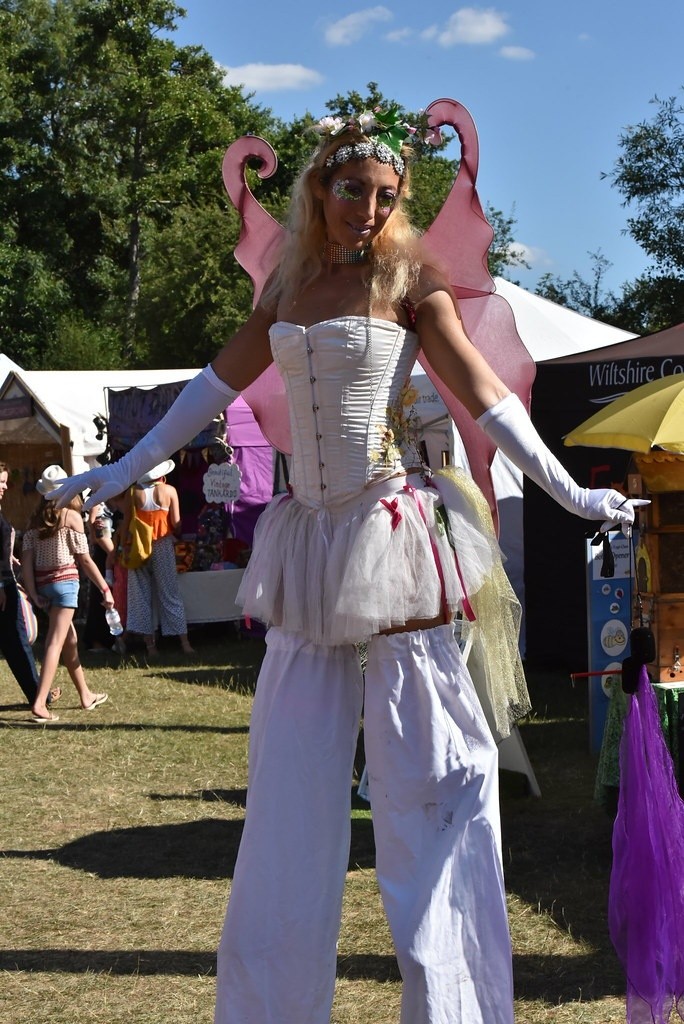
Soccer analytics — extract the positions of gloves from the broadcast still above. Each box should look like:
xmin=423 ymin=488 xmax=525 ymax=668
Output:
xmin=43 ymin=362 xmax=241 ymax=512
xmin=475 ymin=392 xmax=652 ymax=540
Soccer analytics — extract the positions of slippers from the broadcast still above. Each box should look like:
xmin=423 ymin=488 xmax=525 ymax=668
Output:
xmin=32 ymin=713 xmax=60 ymax=723
xmin=82 ymin=692 xmax=108 ymax=710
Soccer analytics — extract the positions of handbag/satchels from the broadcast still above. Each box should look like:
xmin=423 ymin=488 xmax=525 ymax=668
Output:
xmin=119 ymin=485 xmax=152 ymax=569
xmin=9 ymin=526 xmax=38 ymax=645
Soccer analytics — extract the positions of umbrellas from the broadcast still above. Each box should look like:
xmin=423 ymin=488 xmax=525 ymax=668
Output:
xmin=561 ymin=372 xmax=684 ymax=455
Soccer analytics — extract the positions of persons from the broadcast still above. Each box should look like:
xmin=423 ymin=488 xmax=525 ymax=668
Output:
xmin=88 ymin=459 xmax=197 ymax=656
xmin=44 ymin=99 xmax=651 ymax=1023
xmin=22 ymin=465 xmax=114 ymax=723
xmin=0 ymin=462 xmax=63 ymax=708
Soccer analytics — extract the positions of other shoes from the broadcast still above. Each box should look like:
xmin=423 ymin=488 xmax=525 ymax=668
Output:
xmin=49 ymin=687 xmax=63 ymax=703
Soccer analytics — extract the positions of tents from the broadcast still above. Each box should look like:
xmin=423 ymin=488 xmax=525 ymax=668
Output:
xmin=1 ymin=276 xmax=642 ymax=623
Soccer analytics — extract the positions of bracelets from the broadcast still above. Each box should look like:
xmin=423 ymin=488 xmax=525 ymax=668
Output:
xmin=101 ymin=587 xmax=109 ymax=594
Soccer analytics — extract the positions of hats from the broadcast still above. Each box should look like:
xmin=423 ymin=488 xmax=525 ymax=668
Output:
xmin=137 ymin=459 xmax=177 ymax=483
xmin=35 ymin=465 xmax=68 ymax=497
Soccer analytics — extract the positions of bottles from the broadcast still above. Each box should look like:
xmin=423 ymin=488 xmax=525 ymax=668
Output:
xmin=105 ymin=607 xmax=123 ymax=635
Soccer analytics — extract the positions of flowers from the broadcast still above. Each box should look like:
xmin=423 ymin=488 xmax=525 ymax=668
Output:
xmin=316 ymin=82 xmax=444 ymax=155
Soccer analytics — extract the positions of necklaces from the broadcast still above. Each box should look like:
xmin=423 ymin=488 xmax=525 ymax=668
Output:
xmin=326 ymin=243 xmax=370 ymax=263
